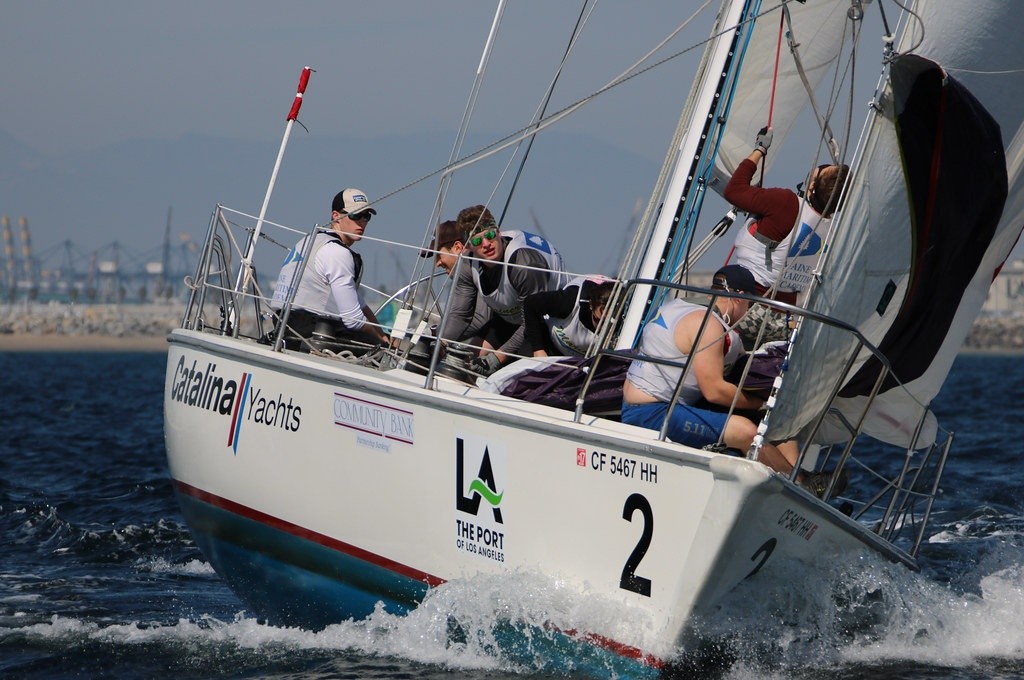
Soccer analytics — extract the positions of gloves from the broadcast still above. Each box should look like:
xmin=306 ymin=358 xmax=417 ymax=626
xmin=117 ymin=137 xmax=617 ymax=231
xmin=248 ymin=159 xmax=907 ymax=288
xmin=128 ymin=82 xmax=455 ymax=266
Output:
xmin=430 ymin=341 xmax=448 ymax=363
xmin=469 ymin=352 xmax=502 ymax=376
xmin=753 ymin=126 xmax=773 ymax=156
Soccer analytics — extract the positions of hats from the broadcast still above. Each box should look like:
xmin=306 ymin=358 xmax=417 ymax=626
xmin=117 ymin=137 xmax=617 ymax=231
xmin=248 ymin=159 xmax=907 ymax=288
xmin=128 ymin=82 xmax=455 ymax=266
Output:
xmin=418 ymin=220 xmax=463 ymax=258
xmin=332 ymin=188 xmax=377 ymax=216
xmin=713 ymin=265 xmax=756 ymax=295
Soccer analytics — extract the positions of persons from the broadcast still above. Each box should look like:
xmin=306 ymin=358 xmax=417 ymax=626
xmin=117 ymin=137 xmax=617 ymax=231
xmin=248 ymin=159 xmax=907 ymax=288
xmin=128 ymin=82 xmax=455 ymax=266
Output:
xmin=523 ymin=274 xmax=630 ymax=361
xmin=431 ymin=204 xmax=573 ymax=378
xmin=418 ymin=219 xmax=493 ymax=356
xmin=724 ymin=125 xmax=854 ymax=353
xmin=270 ymin=188 xmax=393 ymax=347
xmin=618 ymin=262 xmax=807 ymax=484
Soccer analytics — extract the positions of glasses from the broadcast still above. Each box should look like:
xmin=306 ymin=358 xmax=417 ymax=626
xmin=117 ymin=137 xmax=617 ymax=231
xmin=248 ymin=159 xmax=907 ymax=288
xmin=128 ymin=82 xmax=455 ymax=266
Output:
xmin=471 ymin=229 xmax=496 ymax=247
xmin=816 ymin=164 xmax=831 ymax=179
xmin=341 ymin=211 xmax=371 ymax=222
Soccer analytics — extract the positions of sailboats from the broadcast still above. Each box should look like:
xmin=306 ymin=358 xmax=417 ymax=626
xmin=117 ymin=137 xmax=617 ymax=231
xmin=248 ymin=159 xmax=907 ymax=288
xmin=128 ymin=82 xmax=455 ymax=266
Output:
xmin=164 ymin=0 xmax=1024 ymax=680
